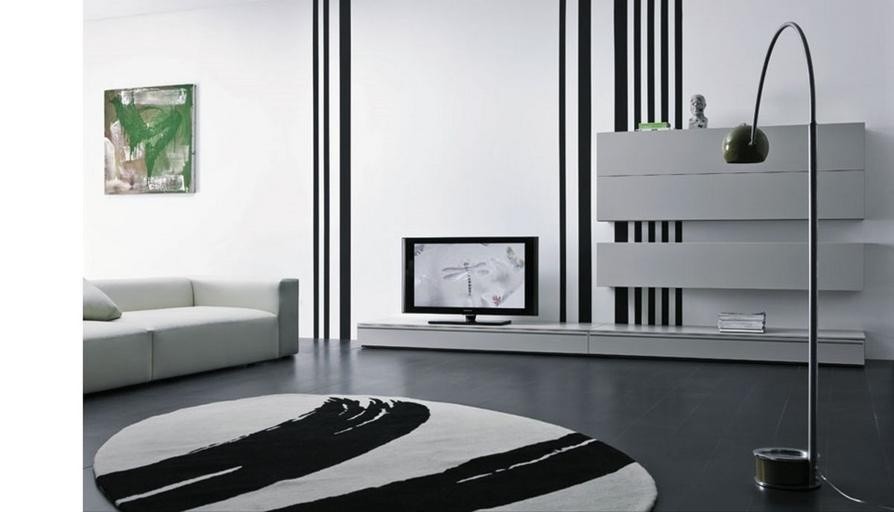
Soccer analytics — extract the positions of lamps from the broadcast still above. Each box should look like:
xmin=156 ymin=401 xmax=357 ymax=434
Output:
xmin=723 ymin=19 xmax=816 ymax=491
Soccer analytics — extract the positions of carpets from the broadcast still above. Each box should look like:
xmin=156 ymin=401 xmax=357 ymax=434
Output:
xmin=92 ymin=393 xmax=661 ymax=511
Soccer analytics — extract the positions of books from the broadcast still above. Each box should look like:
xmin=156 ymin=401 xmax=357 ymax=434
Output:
xmin=715 ymin=311 xmax=766 ymax=334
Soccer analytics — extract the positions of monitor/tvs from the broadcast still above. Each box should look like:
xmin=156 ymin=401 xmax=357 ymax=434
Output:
xmin=400 ymin=235 xmax=540 ymax=325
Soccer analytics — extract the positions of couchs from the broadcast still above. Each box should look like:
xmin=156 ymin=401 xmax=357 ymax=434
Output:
xmin=84 ymin=273 xmax=298 ymax=394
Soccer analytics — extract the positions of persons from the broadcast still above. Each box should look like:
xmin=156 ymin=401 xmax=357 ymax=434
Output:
xmin=687 ymin=95 xmax=708 ymax=128
